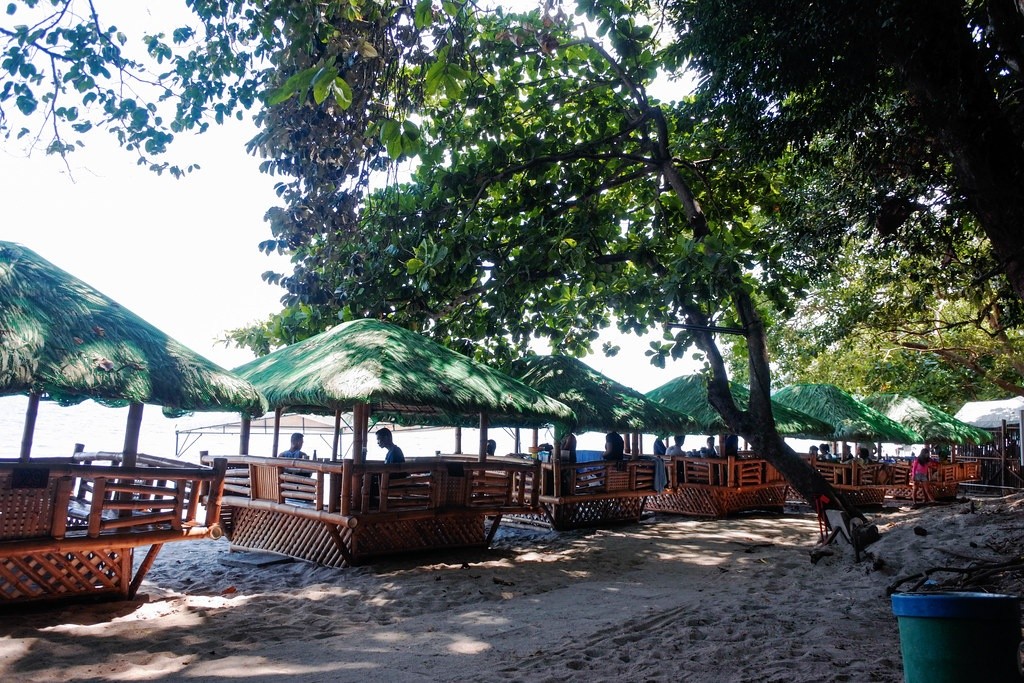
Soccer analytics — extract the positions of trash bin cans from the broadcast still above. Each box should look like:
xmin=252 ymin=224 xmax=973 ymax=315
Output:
xmin=890 ymin=591 xmax=1024 ymax=683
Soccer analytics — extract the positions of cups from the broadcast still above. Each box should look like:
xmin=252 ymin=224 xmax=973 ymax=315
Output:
xmin=536 ymin=451 xmax=544 ymax=462
xmin=692 ymin=449 xmax=696 ymax=455
xmin=317 ymin=458 xmax=323 ymax=463
xmin=323 ymin=458 xmax=330 ymax=464
xmin=532 ymin=452 xmax=537 ymax=459
xmin=542 ymin=451 xmax=550 ymax=463
xmin=302 ymin=455 xmax=309 ymax=460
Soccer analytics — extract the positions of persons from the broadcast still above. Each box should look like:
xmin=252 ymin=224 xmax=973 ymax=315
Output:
xmin=368 ymin=427 xmax=408 ymax=510
xmin=910 ymin=447 xmax=938 ymax=506
xmin=485 ymin=439 xmax=497 ymax=456
xmin=724 ymin=434 xmax=739 ymax=459
xmin=919 ymin=448 xmax=938 ymax=505
xmin=601 ymin=429 xmax=624 ymax=460
xmin=559 ymin=432 xmax=577 ymax=464
xmin=705 ymin=436 xmax=720 ymax=459
xmin=935 ymin=445 xmax=952 ymax=464
xmin=665 ymin=435 xmax=686 ymax=456
xmin=806 ymin=437 xmax=879 ymax=465
xmin=653 ymin=436 xmax=667 ymax=456
xmin=932 ymin=451 xmax=949 ymax=482
xmin=277 ymin=432 xmax=310 ymax=475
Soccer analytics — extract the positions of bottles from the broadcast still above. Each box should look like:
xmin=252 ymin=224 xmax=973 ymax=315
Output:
xmin=312 ymin=450 xmax=317 ymax=461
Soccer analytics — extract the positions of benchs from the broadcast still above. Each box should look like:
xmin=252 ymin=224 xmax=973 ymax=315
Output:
xmin=67 ymin=506 xmax=108 ymax=528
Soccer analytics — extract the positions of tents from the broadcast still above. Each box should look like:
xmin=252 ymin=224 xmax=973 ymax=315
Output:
xmin=339 ymin=410 xmax=522 ymax=461
xmin=173 ymin=410 xmax=342 ymax=461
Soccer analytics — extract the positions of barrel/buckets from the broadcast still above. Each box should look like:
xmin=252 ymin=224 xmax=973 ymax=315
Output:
xmin=891 ymin=591 xmax=1022 ymax=683
